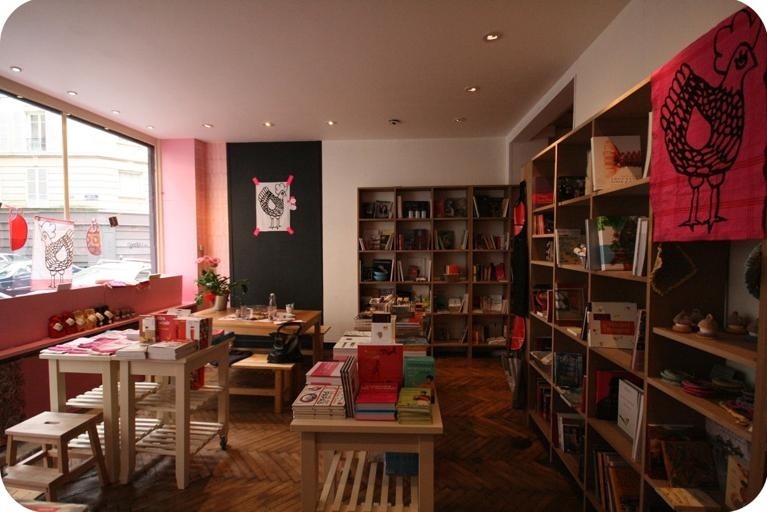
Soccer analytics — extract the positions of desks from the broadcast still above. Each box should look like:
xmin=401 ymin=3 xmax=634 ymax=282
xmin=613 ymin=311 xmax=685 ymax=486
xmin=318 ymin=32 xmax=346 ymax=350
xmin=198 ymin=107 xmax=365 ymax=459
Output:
xmin=39 ymin=331 xmax=120 ymax=483
xmin=190 ymin=304 xmax=323 ymax=386
xmin=292 ymin=325 xmax=441 ymax=511
xmin=118 ymin=331 xmax=234 ymax=490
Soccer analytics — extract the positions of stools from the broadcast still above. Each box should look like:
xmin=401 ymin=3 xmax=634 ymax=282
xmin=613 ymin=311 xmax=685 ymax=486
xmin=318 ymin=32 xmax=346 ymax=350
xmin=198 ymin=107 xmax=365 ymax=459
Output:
xmin=227 ymin=352 xmax=296 ymax=414
xmin=5 ymin=411 xmax=107 ymax=511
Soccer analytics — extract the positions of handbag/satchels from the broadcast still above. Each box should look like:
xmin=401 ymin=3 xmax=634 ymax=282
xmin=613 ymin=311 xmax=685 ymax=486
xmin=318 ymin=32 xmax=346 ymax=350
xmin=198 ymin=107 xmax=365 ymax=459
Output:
xmin=267 ymin=332 xmax=304 ymax=364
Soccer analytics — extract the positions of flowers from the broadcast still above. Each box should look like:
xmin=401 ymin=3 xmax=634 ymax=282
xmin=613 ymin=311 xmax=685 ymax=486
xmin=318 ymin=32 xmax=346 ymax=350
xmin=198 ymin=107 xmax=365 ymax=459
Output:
xmin=192 ymin=255 xmax=250 ymax=309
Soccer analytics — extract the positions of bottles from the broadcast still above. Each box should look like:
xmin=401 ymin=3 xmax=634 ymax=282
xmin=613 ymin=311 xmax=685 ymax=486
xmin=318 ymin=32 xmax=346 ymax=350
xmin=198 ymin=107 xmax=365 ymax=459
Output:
xmin=399 ymin=230 xmax=426 ymax=250
xmin=234 ymin=292 xmax=296 ymax=325
xmin=47 ymin=304 xmax=135 ymax=336
xmin=409 ymin=207 xmax=427 ymax=218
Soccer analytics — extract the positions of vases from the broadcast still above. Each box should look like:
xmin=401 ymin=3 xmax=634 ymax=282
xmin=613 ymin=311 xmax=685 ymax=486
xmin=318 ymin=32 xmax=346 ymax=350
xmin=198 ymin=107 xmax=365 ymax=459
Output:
xmin=213 ymin=293 xmax=229 ymax=310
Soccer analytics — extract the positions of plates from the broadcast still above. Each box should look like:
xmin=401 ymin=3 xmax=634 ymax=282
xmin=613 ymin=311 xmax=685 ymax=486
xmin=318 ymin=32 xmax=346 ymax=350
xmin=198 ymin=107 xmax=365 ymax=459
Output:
xmin=658 ymin=364 xmax=755 ymax=406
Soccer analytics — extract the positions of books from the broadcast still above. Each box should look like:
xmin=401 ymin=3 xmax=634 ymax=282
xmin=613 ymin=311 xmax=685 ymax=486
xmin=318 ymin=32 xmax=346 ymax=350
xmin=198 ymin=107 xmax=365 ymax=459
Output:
xmin=586 ymin=302 xmax=636 ymax=348
xmin=585 ymin=219 xmax=601 ymax=271
xmin=706 ymin=421 xmax=753 ymax=508
xmin=473 ymin=232 xmax=496 ymax=249
xmin=472 ymin=197 xmax=479 ymax=218
xmin=595 ymin=371 xmax=623 ymax=421
xmin=554 ymin=352 xmax=582 ymax=388
xmin=473 ymin=324 xmax=490 ymax=344
xmin=547 ymin=290 xmax=553 ymax=323
xmin=473 ymin=264 xmax=494 ymax=282
xmin=533 ymin=213 xmax=553 ymax=234
xmin=648 ymin=424 xmax=713 ymax=488
xmin=643 ymin=113 xmax=653 ymax=179
xmin=287 ymin=327 xmax=438 ymax=427
xmin=633 ymin=218 xmax=649 ymax=276
xmin=389 ymin=258 xmax=404 ymax=283
xmin=617 ymin=379 xmax=644 ymax=462
xmin=597 ymin=215 xmax=640 ymax=271
xmin=591 ymin=136 xmax=642 ymax=191
xmin=456 ymin=320 xmax=469 ymax=344
xmin=434 ymin=229 xmax=454 ymax=250
xmin=353 ymin=195 xmax=513 ymax=344
xmin=554 ymin=288 xmax=585 ymax=327
xmin=591 ymin=452 xmax=640 ymax=510
xmin=39 ymin=305 xmax=235 ymax=362
xmin=458 ymin=293 xmax=469 ymax=313
xmin=397 ymin=197 xmax=402 ymax=218
xmin=557 ymin=414 xmax=586 ymax=454
xmin=359 ymin=228 xmax=395 ymax=250
xmin=397 ymin=228 xmax=431 ymax=249
xmin=460 ymin=230 xmax=468 ymax=250
xmin=631 ymin=309 xmax=645 ymax=371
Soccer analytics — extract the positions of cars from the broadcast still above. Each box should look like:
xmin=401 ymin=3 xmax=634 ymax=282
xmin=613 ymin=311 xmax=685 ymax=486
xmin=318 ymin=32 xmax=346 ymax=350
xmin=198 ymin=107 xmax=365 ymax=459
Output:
xmin=0 ymin=252 xmax=153 ymax=297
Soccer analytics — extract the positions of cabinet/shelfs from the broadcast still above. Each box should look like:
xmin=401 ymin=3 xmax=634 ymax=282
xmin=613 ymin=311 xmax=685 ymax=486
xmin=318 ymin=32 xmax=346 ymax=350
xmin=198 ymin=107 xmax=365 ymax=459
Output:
xmin=356 ymin=185 xmax=522 ymax=357
xmin=524 ymin=5 xmax=767 ymax=511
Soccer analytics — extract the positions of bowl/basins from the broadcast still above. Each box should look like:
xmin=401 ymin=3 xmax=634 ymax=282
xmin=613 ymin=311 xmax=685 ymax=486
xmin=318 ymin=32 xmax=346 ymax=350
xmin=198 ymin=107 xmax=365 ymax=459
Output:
xmin=374 ymin=272 xmax=386 ymax=282
